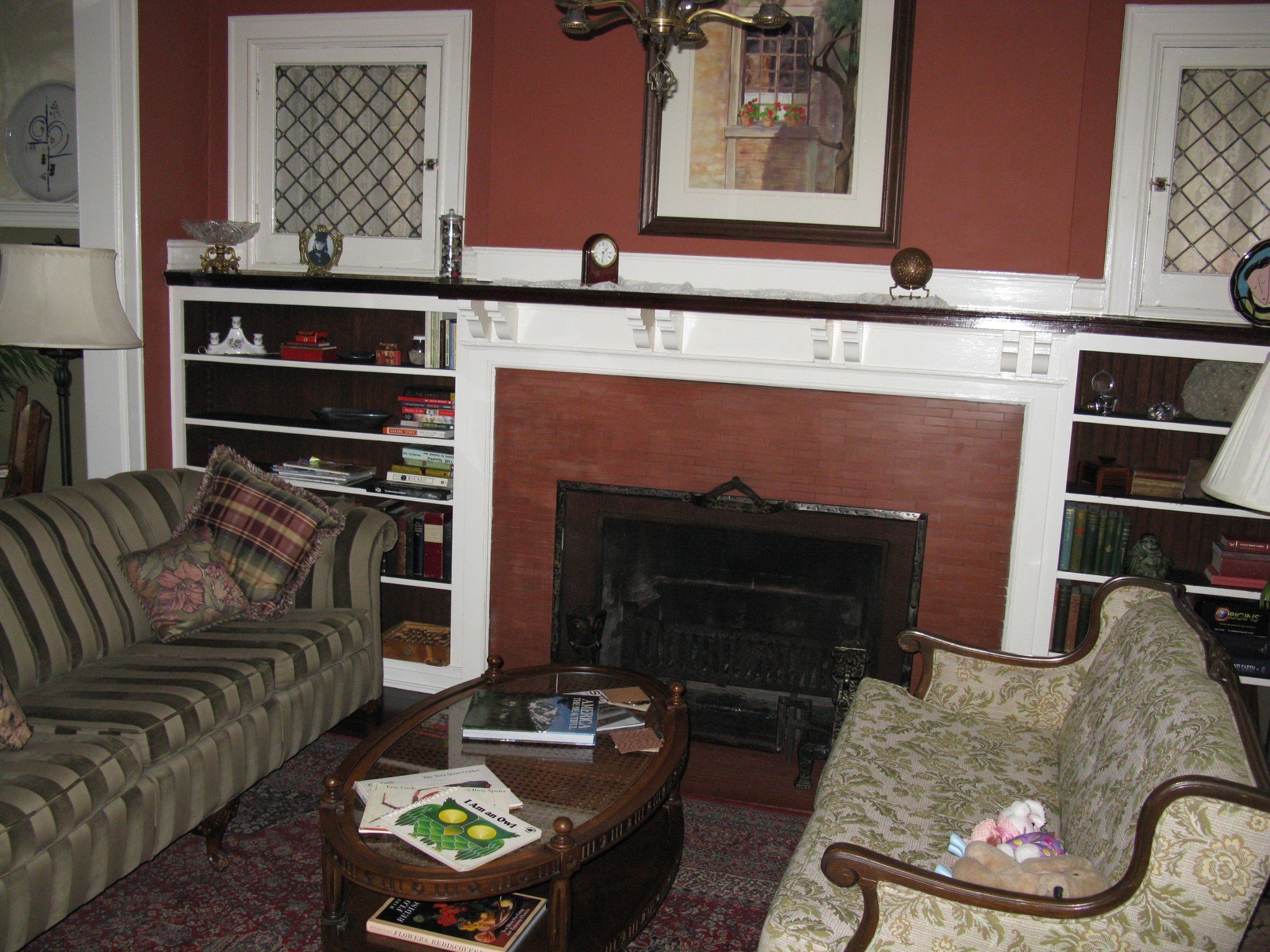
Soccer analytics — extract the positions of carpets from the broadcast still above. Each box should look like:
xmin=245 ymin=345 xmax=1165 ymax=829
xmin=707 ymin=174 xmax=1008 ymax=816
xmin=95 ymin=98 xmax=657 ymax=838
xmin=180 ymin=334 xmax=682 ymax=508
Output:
xmin=27 ymin=729 xmax=824 ymax=951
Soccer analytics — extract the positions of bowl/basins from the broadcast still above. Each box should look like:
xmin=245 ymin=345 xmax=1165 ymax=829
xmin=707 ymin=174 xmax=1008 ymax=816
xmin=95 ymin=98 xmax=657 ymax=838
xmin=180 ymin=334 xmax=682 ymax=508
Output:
xmin=310 ymin=405 xmax=389 ymax=429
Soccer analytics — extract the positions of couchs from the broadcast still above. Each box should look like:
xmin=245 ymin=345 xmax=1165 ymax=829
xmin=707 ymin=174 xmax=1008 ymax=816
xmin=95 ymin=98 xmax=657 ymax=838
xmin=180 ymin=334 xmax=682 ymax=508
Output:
xmin=747 ymin=579 xmax=1269 ymax=950
xmin=0 ymin=470 xmax=402 ymax=952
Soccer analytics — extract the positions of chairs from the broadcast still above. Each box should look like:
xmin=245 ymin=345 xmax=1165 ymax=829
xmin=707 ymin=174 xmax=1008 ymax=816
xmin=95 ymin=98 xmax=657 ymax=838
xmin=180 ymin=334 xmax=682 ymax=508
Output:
xmin=0 ymin=385 xmax=52 ymax=501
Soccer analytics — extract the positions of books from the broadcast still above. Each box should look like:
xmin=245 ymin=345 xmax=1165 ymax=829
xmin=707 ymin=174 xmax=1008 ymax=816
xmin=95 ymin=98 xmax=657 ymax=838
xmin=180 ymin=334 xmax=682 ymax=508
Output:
xmin=1047 ymin=444 xmax=1270 ymax=680
xmin=331 ymin=683 xmax=672 ymax=952
xmin=248 ymin=312 xmax=457 ymax=586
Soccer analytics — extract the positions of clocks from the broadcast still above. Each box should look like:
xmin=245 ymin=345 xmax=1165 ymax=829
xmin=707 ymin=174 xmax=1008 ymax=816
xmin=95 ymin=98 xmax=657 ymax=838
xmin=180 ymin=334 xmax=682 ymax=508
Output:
xmin=580 ymin=232 xmax=618 ymax=289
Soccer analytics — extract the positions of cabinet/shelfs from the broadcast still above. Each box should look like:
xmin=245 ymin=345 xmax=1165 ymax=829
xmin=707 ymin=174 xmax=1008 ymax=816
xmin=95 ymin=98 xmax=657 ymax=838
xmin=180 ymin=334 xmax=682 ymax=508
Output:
xmin=1038 ymin=346 xmax=1269 ymax=686
xmin=168 ymin=285 xmax=494 ymax=694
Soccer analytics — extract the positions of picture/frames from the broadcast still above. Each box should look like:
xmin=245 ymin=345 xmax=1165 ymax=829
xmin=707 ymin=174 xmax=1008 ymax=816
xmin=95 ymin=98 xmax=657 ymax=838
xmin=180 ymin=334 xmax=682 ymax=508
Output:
xmin=297 ymin=225 xmax=344 ymax=276
xmin=639 ymin=0 xmax=914 ymax=247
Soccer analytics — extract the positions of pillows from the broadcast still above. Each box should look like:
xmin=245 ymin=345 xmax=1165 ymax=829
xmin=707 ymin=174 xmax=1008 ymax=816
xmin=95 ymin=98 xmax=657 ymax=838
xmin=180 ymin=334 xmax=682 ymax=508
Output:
xmin=115 ymin=522 xmax=257 ymax=641
xmin=173 ymin=443 xmax=346 ymax=623
xmin=1 ymin=667 xmax=39 ymax=751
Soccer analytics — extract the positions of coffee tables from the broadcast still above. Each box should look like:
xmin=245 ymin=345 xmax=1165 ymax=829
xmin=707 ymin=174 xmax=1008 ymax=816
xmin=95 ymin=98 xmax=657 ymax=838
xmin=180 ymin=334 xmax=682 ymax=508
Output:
xmin=319 ymin=655 xmax=690 ymax=952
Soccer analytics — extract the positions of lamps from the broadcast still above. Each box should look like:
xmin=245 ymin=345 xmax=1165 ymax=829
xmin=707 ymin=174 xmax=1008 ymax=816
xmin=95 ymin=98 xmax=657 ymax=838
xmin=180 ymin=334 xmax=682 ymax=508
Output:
xmin=555 ymin=0 xmax=791 ymax=110
xmin=0 ymin=242 xmax=147 ymax=486
xmin=1201 ymin=358 xmax=1268 ymax=520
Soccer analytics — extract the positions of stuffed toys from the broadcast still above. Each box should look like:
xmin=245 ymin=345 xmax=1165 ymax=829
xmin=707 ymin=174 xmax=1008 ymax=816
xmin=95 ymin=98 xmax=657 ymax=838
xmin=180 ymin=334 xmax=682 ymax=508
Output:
xmin=931 ymin=794 xmax=1116 ymax=898
